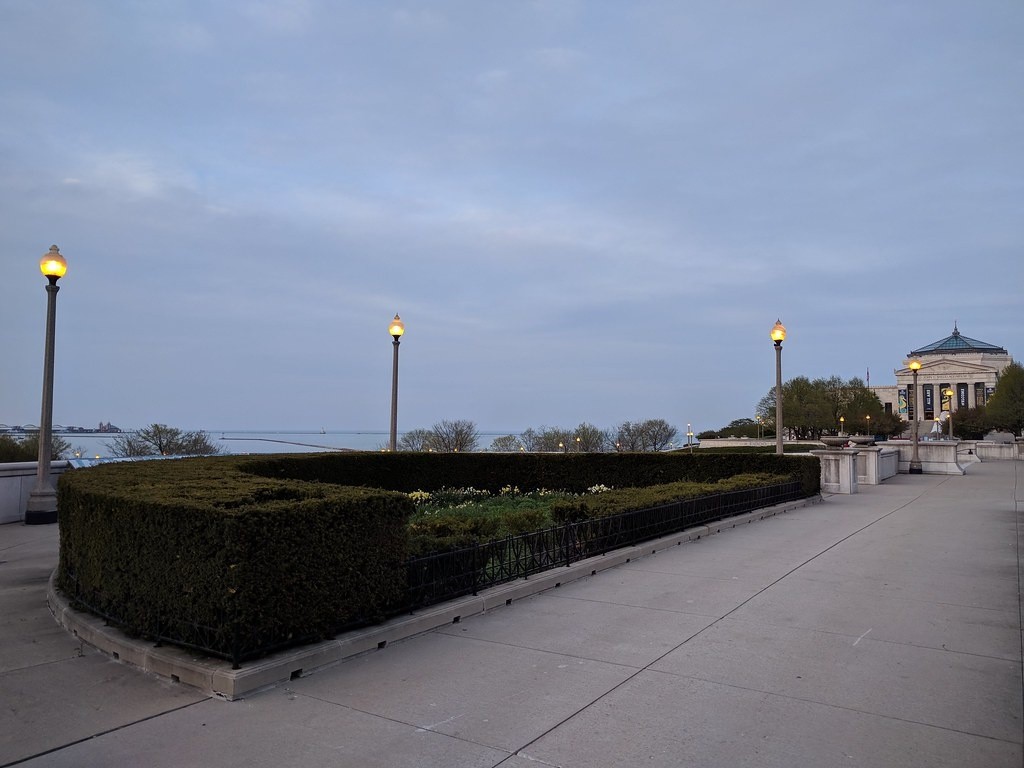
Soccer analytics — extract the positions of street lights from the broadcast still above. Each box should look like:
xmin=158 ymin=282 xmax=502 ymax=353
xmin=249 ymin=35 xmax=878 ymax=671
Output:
xmin=866 ymin=414 xmax=870 ymax=435
xmin=24 ymin=244 xmax=68 ymax=525
xmin=840 ymin=416 xmax=844 ymax=436
xmin=388 ymin=313 xmax=405 ymax=451
xmin=687 ymin=430 xmax=693 ymax=453
xmin=946 ymin=390 xmax=954 ymax=440
xmin=934 ymin=416 xmax=940 ymax=432
xmin=770 ymin=318 xmax=787 ymax=454
xmin=756 ymin=414 xmax=764 ymax=440
xmin=908 ymin=355 xmax=922 ymax=474
xmin=577 ymin=438 xmax=580 ymax=452
